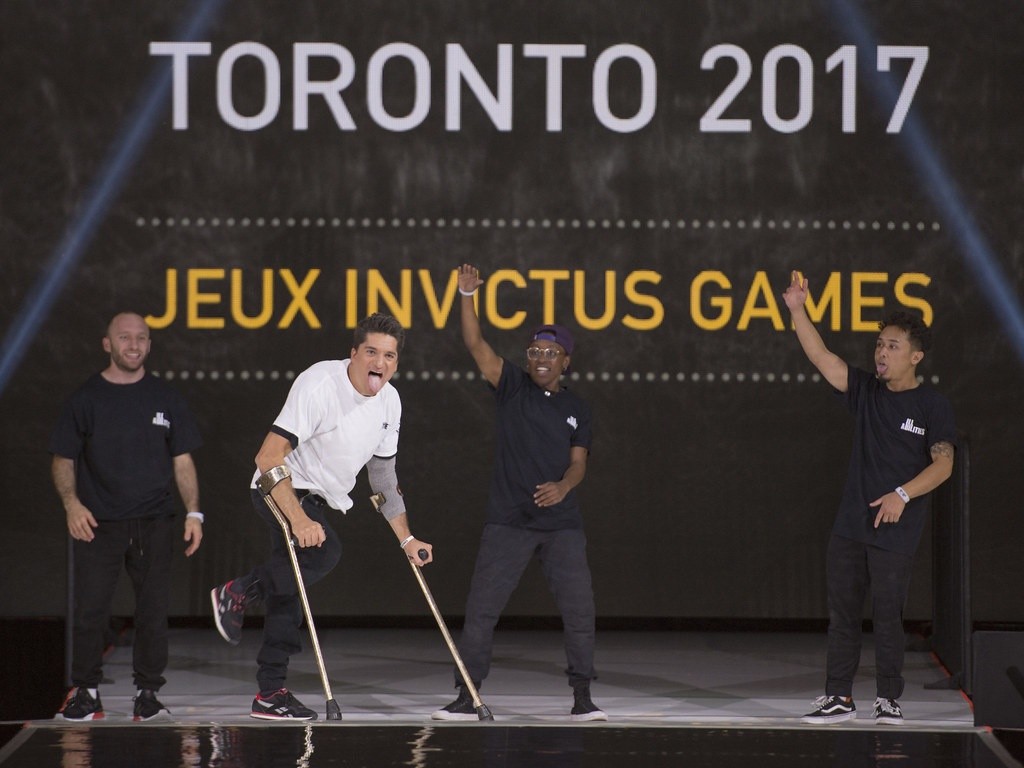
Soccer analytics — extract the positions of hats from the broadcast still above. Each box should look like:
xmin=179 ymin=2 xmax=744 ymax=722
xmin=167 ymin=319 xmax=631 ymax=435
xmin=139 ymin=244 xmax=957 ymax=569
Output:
xmin=528 ymin=325 xmax=575 ymax=376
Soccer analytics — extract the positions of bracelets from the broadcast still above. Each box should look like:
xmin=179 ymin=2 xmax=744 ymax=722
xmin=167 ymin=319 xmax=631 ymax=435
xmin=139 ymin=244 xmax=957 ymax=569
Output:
xmin=186 ymin=512 xmax=204 ymax=523
xmin=895 ymin=487 xmax=910 ymax=503
xmin=400 ymin=535 xmax=415 ymax=549
xmin=458 ymin=287 xmax=475 ymax=296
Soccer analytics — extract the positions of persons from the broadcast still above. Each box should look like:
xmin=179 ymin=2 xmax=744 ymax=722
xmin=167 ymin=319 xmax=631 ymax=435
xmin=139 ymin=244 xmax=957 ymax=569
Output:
xmin=211 ymin=313 xmax=433 ymax=721
xmin=784 ymin=271 xmax=955 ymax=725
xmin=48 ymin=311 xmax=204 ymax=723
xmin=431 ymin=263 xmax=609 ymax=721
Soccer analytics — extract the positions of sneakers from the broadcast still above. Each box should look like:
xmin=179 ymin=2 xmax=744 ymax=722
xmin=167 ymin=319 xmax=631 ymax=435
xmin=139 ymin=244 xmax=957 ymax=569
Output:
xmin=431 ymin=684 xmax=481 ymax=721
xmin=870 ymin=697 xmax=904 ymax=726
xmin=250 ymin=688 xmax=318 ymax=720
xmin=210 ymin=578 xmax=264 ymax=647
xmin=132 ymin=688 xmax=173 ymax=721
xmin=570 ymin=687 xmax=608 ymax=721
xmin=800 ymin=696 xmax=856 ymax=725
xmin=55 ymin=688 xmax=105 ymax=722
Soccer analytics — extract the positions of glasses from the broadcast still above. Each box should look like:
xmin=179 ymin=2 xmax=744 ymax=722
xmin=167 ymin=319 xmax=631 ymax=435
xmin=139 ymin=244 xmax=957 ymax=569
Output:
xmin=526 ymin=346 xmax=565 ymax=361
